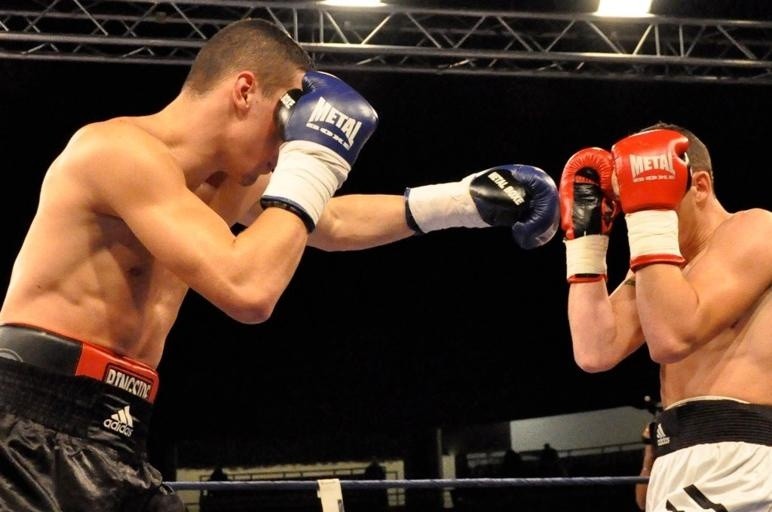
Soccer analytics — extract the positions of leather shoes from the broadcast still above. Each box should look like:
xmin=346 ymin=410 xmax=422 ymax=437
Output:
xmin=403 ymin=161 xmax=562 ymax=252
xmin=256 ymin=69 xmax=381 ymax=233
xmin=612 ymin=127 xmax=688 ymax=269
xmin=561 ymin=146 xmax=621 ymax=284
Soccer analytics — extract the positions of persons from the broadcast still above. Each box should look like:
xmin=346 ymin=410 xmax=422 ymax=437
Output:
xmin=499 ymin=448 xmax=521 ymax=468
xmin=560 ymin=122 xmax=772 ymax=512
xmin=364 ymin=456 xmax=388 ymax=479
xmin=0 ymin=17 xmax=562 ymax=510
xmin=209 ymin=463 xmax=227 ymax=481
